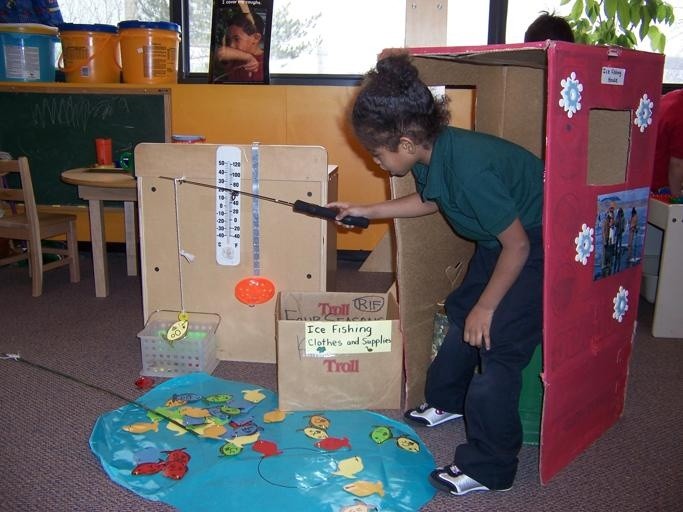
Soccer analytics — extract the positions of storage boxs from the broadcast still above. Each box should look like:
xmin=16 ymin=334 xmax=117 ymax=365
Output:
xmin=275 ymin=290 xmax=405 ymax=413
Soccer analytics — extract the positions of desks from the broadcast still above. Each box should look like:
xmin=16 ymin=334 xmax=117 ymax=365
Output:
xmin=646 ymin=196 xmax=681 ymax=340
xmin=61 ymin=166 xmax=143 ymax=298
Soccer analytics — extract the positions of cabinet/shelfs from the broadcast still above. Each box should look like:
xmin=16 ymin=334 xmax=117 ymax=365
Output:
xmin=168 ymin=83 xmax=476 ymax=262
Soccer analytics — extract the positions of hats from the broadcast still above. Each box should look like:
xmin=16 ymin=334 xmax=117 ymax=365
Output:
xmin=610 ymin=202 xmax=617 ymax=208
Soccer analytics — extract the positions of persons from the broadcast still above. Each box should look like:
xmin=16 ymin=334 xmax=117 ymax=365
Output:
xmin=217 ymin=13 xmax=264 ymax=82
xmin=651 ymin=90 xmax=682 ymax=201
xmin=525 ymin=10 xmax=574 ymax=45
xmin=325 ymin=53 xmax=544 ymax=497
xmin=600 ymin=202 xmax=637 ymax=248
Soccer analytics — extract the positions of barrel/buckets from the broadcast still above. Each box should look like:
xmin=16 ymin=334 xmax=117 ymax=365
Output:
xmin=0 ymin=23 xmax=57 ymax=83
xmin=59 ymin=22 xmax=119 ymax=84
xmin=118 ymin=20 xmax=181 ymax=84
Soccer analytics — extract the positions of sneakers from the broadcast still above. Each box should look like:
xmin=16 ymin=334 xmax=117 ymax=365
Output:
xmin=428 ymin=462 xmax=513 ymax=496
xmin=403 ymin=402 xmax=463 ymax=427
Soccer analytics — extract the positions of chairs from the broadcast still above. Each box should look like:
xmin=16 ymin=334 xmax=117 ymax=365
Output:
xmin=0 ymin=154 xmax=81 ymax=298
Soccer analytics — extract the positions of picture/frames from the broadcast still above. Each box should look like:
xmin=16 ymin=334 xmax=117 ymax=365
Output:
xmin=207 ymin=1 xmax=274 ymax=86
